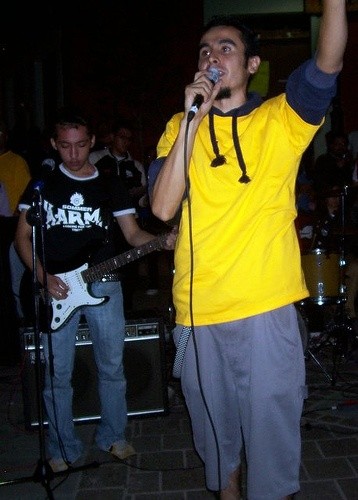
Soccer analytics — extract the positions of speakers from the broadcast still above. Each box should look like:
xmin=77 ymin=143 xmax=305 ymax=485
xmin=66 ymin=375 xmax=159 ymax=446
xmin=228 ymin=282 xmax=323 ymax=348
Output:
xmin=18 ymin=316 xmax=169 ymax=431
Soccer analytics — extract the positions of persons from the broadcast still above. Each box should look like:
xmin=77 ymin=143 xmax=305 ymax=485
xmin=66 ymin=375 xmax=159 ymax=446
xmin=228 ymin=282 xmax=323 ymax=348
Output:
xmin=14 ymin=112 xmax=180 ymax=477
xmin=295 ymin=127 xmax=358 ymax=319
xmin=0 ymin=123 xmax=174 ymax=367
xmin=147 ymin=0 xmax=347 ymax=500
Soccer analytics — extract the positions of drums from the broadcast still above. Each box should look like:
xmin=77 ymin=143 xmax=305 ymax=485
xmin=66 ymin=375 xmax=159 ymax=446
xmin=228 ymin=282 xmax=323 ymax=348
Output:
xmin=296 ymin=247 xmax=348 ymax=307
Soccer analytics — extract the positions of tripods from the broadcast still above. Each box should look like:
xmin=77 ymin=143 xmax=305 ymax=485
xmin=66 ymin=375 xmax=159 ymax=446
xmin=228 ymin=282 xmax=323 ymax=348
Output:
xmin=0 ymin=195 xmax=98 ymax=500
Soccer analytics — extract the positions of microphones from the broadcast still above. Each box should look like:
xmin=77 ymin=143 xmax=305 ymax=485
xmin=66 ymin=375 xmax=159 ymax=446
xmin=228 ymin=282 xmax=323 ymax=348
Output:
xmin=32 ymin=159 xmax=55 ymax=198
xmin=186 ymin=67 xmax=219 ymax=123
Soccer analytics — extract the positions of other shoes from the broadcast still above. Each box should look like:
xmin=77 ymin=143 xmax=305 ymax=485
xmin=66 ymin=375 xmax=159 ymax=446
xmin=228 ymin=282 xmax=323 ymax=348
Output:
xmin=111 ymin=440 xmax=136 ymax=460
xmin=43 ymin=457 xmax=73 ymax=471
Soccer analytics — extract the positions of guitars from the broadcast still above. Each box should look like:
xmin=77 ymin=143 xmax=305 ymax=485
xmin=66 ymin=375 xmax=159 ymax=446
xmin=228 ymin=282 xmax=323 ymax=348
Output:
xmin=19 ymin=229 xmax=180 ymax=334
